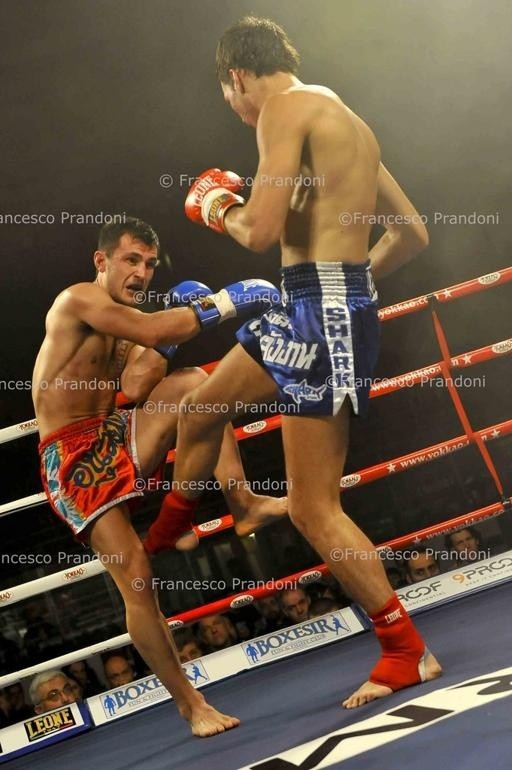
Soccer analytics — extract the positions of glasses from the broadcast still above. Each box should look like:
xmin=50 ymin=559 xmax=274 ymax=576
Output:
xmin=33 ymin=682 xmax=75 ymax=708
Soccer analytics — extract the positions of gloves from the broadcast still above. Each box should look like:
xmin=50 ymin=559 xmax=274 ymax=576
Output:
xmin=183 ymin=166 xmax=249 ymax=237
xmin=151 ymin=278 xmax=214 ymax=362
xmin=189 ymin=277 xmax=284 ymax=331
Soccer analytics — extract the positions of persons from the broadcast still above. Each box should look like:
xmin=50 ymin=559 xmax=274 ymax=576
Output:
xmin=0 ymin=527 xmax=489 ymax=727
xmin=35 ymin=213 xmax=289 ymax=738
xmin=139 ymin=14 xmax=442 ymax=710
